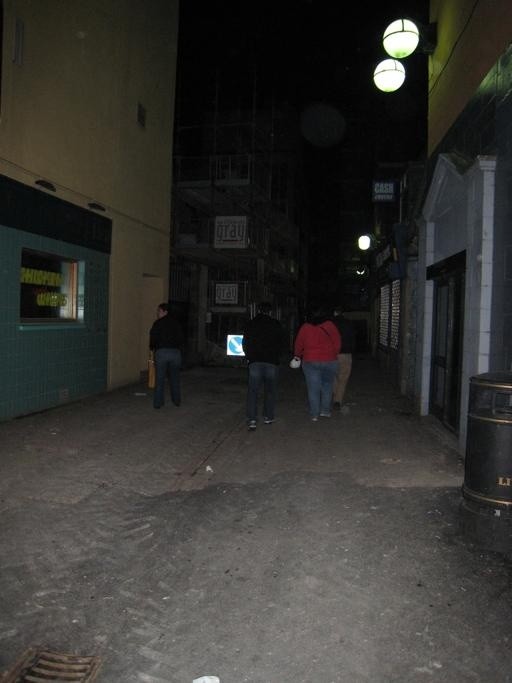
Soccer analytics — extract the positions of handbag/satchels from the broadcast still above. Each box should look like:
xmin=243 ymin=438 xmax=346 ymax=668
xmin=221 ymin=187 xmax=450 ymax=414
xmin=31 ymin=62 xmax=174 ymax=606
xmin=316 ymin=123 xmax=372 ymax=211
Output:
xmin=290 ymin=356 xmax=301 ymax=368
xmin=148 ymin=358 xmax=157 ymax=389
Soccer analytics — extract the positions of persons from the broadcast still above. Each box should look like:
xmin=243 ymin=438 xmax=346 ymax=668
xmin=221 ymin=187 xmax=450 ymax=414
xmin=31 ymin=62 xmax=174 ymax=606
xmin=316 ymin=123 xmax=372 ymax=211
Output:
xmin=333 ymin=314 xmax=355 ymax=410
xmin=148 ymin=302 xmax=184 ymax=409
xmin=241 ymin=300 xmax=285 ymax=430
xmin=292 ymin=305 xmax=343 ymax=422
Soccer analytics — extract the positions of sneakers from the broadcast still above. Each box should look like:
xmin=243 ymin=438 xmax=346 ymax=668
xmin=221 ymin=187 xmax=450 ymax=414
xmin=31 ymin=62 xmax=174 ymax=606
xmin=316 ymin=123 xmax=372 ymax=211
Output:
xmin=264 ymin=416 xmax=277 ymax=424
xmin=309 ymin=403 xmax=342 ymax=422
xmin=247 ymin=419 xmax=257 ymax=431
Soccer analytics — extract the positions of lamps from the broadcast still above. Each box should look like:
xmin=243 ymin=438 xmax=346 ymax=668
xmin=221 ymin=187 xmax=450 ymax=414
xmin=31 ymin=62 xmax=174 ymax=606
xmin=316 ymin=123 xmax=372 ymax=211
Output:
xmin=382 ymin=17 xmax=435 ymax=59
xmin=356 ymin=264 xmax=365 ymax=275
xmin=358 ymin=235 xmax=380 ymax=251
xmin=372 ymin=58 xmax=406 ymax=92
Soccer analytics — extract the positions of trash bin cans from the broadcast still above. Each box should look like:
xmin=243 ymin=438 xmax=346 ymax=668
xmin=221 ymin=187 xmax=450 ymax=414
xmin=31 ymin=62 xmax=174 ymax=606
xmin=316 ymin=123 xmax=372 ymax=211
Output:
xmin=460 ymin=371 xmax=512 ymax=554
